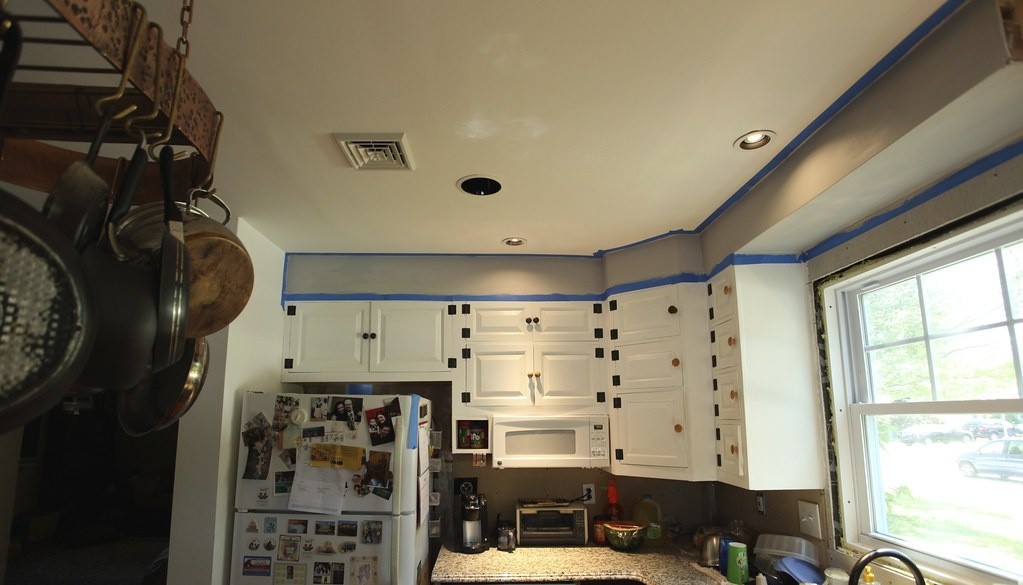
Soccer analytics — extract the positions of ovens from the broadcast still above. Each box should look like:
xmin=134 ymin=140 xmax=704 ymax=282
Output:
xmin=516 ymin=504 xmax=588 ymax=547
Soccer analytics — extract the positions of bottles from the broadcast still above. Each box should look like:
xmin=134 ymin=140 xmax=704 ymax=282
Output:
xmin=632 ymin=495 xmax=664 ymax=548
xmin=604 ymin=479 xmax=624 ymax=521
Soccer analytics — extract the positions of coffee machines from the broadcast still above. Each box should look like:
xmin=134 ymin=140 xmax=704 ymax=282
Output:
xmin=460 ymin=493 xmax=489 ymax=554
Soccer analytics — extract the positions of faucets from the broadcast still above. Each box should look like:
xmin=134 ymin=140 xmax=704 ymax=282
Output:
xmin=849 ymin=547 xmax=925 ymax=585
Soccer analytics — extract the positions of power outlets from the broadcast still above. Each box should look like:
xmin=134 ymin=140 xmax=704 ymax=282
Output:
xmin=583 ymin=483 xmax=595 ymax=504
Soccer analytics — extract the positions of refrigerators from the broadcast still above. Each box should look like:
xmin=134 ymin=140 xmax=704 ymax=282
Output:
xmin=229 ymin=390 xmax=431 ymax=585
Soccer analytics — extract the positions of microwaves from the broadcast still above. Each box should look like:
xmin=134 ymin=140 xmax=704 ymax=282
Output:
xmin=492 ymin=415 xmax=610 ymax=470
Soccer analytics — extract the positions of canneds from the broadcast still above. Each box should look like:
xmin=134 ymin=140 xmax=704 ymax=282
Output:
xmin=458 ymin=420 xmax=485 ymax=449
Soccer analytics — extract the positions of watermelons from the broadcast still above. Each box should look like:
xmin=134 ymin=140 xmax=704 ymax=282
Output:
xmin=602 ymin=522 xmax=647 ymax=550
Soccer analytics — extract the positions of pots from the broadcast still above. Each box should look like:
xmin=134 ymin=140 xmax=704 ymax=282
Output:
xmin=0 ymin=10 xmax=254 ymax=438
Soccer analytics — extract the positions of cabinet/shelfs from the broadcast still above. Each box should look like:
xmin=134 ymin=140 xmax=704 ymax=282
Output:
xmin=280 ymin=300 xmax=453 ymax=382
xmin=452 ymin=301 xmax=609 ymax=417
xmin=705 ymin=253 xmax=825 ymax=490
xmin=599 ymin=281 xmax=705 ymax=482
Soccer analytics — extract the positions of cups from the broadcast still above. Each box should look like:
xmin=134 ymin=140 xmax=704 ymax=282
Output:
xmin=496 ymin=525 xmax=516 ymax=551
xmin=719 ymin=538 xmax=749 ymax=583
